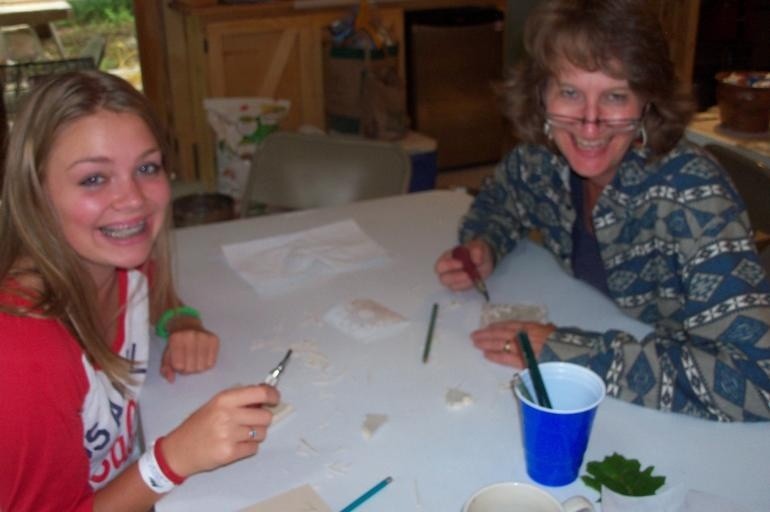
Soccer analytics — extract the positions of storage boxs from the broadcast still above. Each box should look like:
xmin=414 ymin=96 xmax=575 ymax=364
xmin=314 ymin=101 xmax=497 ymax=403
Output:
xmin=399 ymin=130 xmax=439 ymax=192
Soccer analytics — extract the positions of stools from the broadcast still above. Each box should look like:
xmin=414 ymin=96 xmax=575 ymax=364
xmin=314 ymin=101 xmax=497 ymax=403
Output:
xmin=171 ymin=193 xmax=234 ymax=228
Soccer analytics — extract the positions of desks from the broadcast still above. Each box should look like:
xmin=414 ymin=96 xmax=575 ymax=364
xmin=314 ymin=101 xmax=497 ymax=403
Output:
xmin=674 ymin=104 xmax=770 ymax=266
xmin=133 ymin=184 xmax=770 ymax=511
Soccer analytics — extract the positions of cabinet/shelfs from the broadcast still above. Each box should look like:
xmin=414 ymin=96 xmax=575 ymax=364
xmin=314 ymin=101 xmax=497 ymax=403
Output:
xmin=405 ymin=6 xmax=505 ymax=173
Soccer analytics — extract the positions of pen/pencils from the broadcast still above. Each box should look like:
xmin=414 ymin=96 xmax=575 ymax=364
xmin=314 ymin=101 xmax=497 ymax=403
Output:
xmin=514 ymin=373 xmax=535 ymax=403
xmin=340 ymin=477 xmax=392 ymax=512
xmin=423 ymin=303 xmax=439 ymax=362
xmin=516 ymin=331 xmax=552 ymax=409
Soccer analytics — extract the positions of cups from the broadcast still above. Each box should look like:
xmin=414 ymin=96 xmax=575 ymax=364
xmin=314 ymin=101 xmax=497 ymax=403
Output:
xmin=462 ymin=362 xmax=682 ymax=511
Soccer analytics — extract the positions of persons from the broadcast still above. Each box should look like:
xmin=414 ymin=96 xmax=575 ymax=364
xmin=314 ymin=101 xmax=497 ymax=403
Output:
xmin=435 ymin=0 xmax=770 ymax=422
xmin=0 ymin=71 xmax=280 ymax=512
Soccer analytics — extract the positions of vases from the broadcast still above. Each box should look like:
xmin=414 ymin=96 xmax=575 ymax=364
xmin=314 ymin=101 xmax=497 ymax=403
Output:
xmin=714 ymin=69 xmax=770 ymax=132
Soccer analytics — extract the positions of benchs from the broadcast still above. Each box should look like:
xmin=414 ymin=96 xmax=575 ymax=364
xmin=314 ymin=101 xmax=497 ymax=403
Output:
xmin=0 ymin=58 xmax=94 ymax=115
xmin=0 ymin=0 xmax=70 ymax=50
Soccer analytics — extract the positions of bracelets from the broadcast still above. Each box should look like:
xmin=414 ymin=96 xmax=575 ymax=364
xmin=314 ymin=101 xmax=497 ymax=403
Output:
xmin=137 ymin=437 xmax=185 ymax=495
xmin=156 ymin=306 xmax=200 ymax=341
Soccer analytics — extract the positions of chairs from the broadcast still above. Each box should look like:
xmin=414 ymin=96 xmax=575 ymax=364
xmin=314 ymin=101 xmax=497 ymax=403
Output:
xmin=49 ymin=34 xmax=106 ymax=77
xmin=239 ymin=133 xmax=413 ymax=218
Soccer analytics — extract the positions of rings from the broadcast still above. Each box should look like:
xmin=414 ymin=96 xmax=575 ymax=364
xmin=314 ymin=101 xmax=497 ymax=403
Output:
xmin=250 ymin=426 xmax=256 ymax=441
xmin=504 ymin=341 xmax=511 ymax=352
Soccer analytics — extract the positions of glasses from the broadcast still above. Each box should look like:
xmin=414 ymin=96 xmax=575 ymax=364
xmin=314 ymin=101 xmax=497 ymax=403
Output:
xmin=544 ymin=102 xmax=648 ymax=132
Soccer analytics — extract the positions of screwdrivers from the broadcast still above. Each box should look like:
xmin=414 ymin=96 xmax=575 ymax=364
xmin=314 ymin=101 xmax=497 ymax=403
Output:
xmin=246 ymin=349 xmax=294 ymax=410
xmin=452 ymin=246 xmax=490 ymax=302
